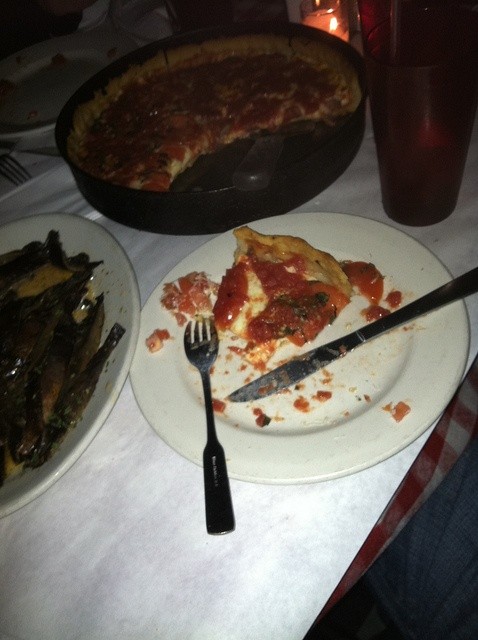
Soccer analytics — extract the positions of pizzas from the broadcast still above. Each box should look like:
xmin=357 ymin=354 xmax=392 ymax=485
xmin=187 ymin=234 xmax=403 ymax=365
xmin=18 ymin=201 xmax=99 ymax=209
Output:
xmin=64 ymin=25 xmax=365 ymax=197
xmin=211 ymin=223 xmax=352 ymax=368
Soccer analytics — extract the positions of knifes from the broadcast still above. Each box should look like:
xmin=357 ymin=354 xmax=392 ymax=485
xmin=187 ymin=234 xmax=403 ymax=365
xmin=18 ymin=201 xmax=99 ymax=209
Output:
xmin=222 ymin=267 xmax=478 ymax=404
xmin=0 ymin=140 xmax=63 ymax=158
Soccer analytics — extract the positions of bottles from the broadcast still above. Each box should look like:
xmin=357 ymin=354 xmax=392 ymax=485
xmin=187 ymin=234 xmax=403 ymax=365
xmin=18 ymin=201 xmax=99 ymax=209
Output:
xmin=360 ymin=16 xmax=478 ymax=227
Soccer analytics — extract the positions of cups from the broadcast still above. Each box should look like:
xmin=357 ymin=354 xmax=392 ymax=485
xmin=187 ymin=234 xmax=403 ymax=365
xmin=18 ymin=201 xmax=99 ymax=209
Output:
xmin=357 ymin=1 xmax=477 ymax=48
xmin=299 ymin=0 xmax=350 ymax=44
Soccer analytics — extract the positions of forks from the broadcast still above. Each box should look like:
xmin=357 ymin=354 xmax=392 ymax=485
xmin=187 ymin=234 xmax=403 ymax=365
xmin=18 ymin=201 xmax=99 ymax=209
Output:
xmin=1 ymin=153 xmax=34 ymax=187
xmin=182 ymin=316 xmax=236 ymax=535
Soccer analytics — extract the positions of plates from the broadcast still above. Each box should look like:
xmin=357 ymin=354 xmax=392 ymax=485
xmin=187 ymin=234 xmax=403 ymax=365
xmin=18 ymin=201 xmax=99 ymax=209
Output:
xmin=127 ymin=213 xmax=471 ymax=485
xmin=1 ymin=28 xmax=142 ymax=139
xmin=0 ymin=212 xmax=141 ymax=520
